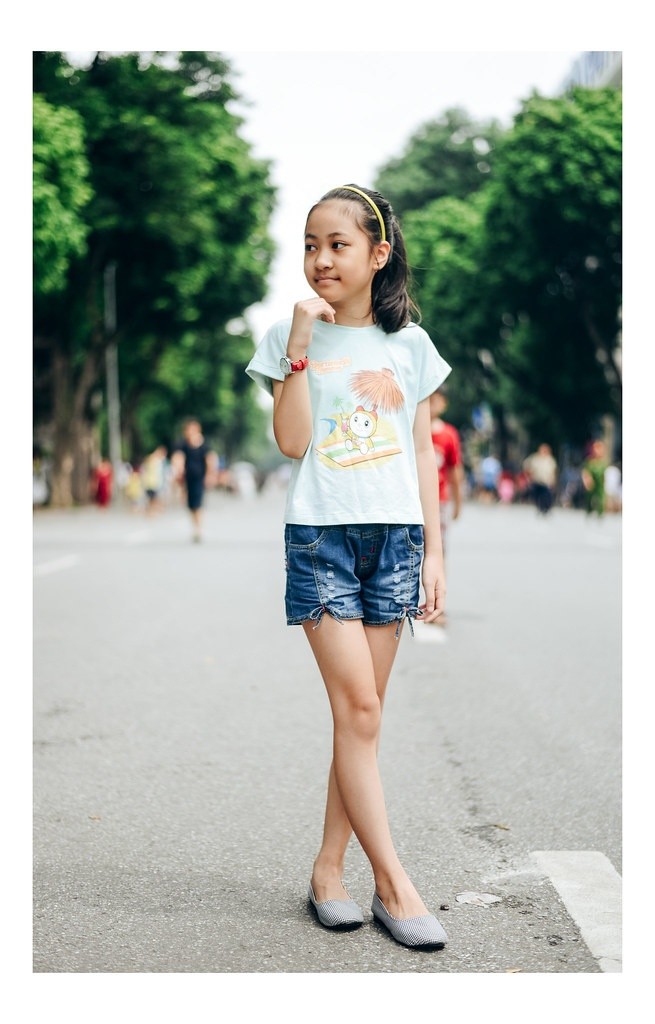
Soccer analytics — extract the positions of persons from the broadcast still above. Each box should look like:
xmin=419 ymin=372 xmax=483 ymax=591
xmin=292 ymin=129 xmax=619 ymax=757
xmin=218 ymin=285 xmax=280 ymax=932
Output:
xmin=98 ymin=416 xmax=255 ymax=553
xmin=430 ymin=391 xmax=463 ymax=564
xmin=245 ymin=183 xmax=453 ymax=948
xmin=463 ymin=438 xmax=623 ymax=520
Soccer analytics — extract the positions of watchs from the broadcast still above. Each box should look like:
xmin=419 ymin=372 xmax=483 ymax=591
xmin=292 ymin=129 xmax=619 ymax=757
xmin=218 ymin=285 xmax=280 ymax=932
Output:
xmin=279 ymin=355 xmax=309 ymax=375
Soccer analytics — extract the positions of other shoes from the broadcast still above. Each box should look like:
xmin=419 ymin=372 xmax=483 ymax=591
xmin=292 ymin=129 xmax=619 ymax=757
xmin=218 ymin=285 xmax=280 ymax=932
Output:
xmin=308 ymin=880 xmax=364 ymax=929
xmin=370 ymin=892 xmax=447 ymax=949
